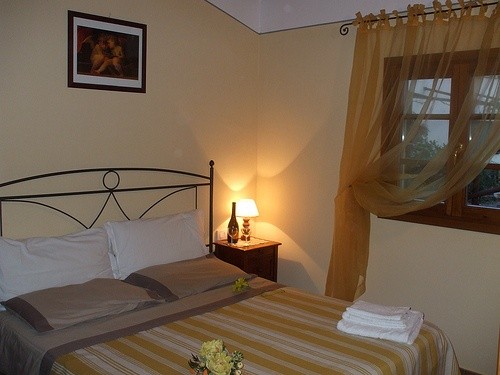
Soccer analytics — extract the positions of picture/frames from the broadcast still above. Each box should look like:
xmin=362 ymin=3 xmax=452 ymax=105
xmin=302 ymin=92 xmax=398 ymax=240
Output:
xmin=68 ymin=9 xmax=147 ymax=93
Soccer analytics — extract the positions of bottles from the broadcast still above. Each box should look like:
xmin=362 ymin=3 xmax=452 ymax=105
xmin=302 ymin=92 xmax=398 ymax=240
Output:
xmin=227 ymin=201 xmax=239 ymax=243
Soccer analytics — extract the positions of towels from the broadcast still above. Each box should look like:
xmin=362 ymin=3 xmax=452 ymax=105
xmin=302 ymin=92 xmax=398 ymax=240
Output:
xmin=337 ymin=299 xmax=424 ymax=345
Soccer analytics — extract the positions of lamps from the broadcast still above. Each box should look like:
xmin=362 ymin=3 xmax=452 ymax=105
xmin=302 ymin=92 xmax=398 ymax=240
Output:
xmin=235 ymin=198 xmax=260 ymax=241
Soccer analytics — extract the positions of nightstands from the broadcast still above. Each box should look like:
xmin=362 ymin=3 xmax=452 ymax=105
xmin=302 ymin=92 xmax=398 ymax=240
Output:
xmin=215 ymin=236 xmax=282 ymax=282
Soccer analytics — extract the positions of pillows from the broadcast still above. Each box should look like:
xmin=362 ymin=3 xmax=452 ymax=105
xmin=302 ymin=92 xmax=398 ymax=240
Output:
xmin=124 ymin=254 xmax=258 ymax=302
xmin=0 ymin=226 xmax=115 ymax=311
xmin=1 ymin=278 xmax=166 ymax=335
xmin=104 ymin=209 xmax=208 ymax=280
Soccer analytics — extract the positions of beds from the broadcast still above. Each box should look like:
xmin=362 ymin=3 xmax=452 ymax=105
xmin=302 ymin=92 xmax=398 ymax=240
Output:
xmin=0 ymin=160 xmax=463 ymax=375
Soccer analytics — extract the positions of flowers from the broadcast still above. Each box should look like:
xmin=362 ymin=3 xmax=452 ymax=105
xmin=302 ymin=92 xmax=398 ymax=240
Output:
xmin=188 ymin=339 xmax=244 ymax=375
xmin=232 ymin=277 xmax=254 ymax=294
